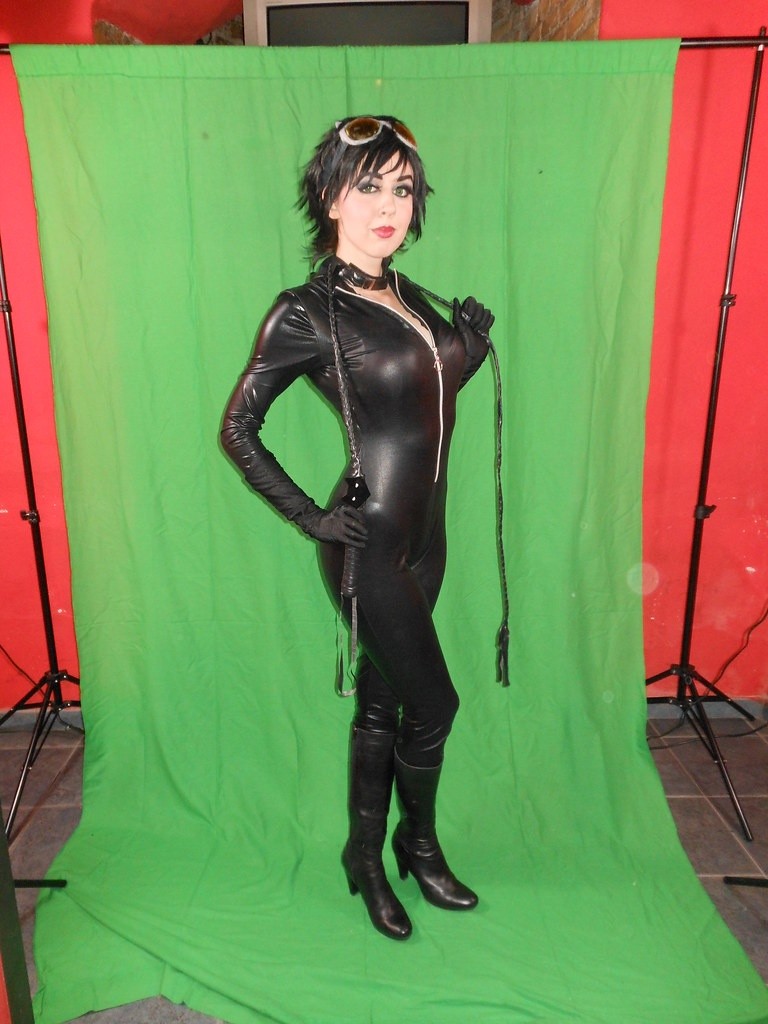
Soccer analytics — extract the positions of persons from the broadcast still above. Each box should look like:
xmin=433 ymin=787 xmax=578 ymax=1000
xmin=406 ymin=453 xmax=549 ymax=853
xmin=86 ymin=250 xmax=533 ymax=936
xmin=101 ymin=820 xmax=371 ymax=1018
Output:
xmin=220 ymin=115 xmax=509 ymax=941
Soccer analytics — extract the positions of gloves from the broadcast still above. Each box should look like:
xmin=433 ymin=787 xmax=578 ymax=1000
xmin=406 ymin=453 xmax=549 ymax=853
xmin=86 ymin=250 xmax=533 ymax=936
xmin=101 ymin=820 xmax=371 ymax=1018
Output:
xmin=453 ymin=296 xmax=495 ymax=361
xmin=293 ymin=504 xmax=369 ymax=550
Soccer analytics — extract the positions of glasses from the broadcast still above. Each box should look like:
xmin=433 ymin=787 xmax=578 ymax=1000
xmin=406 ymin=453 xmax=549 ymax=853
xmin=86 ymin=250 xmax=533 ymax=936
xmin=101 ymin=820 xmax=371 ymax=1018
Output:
xmin=326 ymin=117 xmax=418 ymax=184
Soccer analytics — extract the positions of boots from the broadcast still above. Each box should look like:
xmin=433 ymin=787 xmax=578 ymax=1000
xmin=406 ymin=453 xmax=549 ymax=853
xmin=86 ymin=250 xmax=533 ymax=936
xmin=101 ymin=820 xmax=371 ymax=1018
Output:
xmin=392 ymin=744 xmax=478 ymax=911
xmin=341 ymin=724 xmax=413 ymax=941
